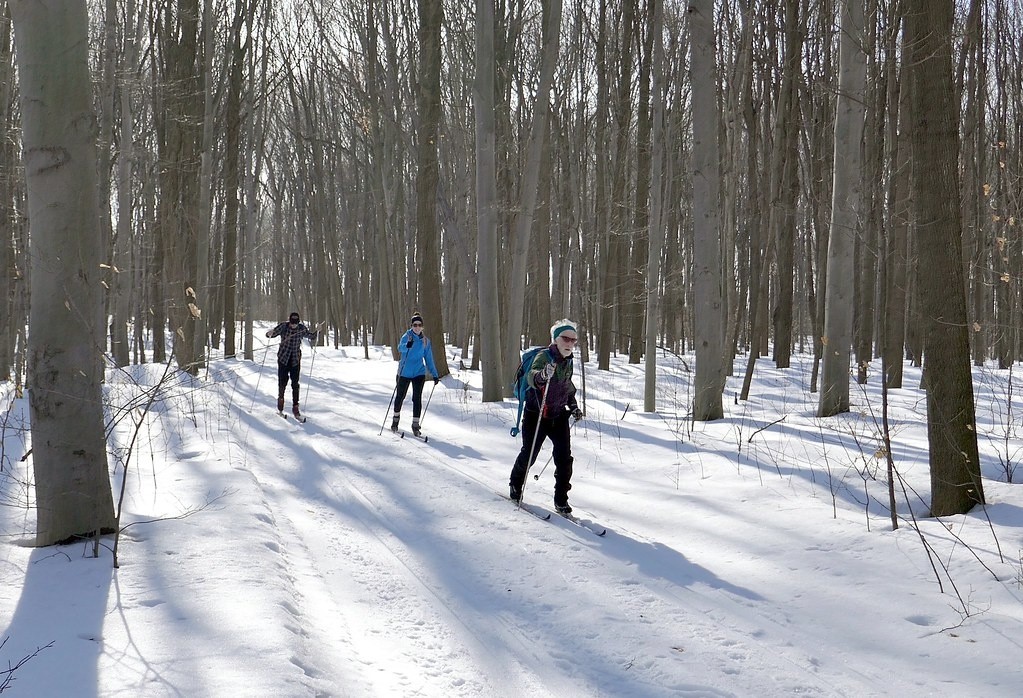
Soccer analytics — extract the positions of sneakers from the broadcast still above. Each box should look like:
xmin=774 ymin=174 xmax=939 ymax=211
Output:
xmin=293 ymin=403 xmax=300 ymax=416
xmin=391 ymin=415 xmax=400 ymax=431
xmin=554 ymin=491 xmax=572 ymax=513
xmin=278 ymin=398 xmax=284 ymax=411
xmin=509 ymin=478 xmax=523 ymax=499
xmin=412 ymin=421 xmax=421 ymax=436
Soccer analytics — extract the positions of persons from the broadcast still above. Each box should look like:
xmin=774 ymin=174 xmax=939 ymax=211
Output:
xmin=508 ymin=319 xmax=583 ymax=516
xmin=391 ymin=312 xmax=439 ymax=437
xmin=266 ymin=313 xmax=326 ymax=416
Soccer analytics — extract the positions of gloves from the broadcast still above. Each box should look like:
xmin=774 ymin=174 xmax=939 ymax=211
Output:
xmin=540 ymin=363 xmax=555 ymax=380
xmin=433 ymin=377 xmax=438 ymax=385
xmin=406 ymin=338 xmax=414 ymax=348
xmin=569 ymin=403 xmax=583 ymax=423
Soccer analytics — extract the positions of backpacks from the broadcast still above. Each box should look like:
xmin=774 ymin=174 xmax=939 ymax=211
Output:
xmin=510 ymin=348 xmax=548 ymax=437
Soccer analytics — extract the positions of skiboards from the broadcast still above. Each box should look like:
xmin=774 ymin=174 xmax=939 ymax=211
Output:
xmin=392 ymin=430 xmax=429 ymax=444
xmin=275 ymin=411 xmax=307 ymax=423
xmin=495 ymin=491 xmax=607 ymax=537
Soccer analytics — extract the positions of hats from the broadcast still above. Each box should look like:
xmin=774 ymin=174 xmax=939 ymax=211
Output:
xmin=412 ymin=312 xmax=423 ymax=325
xmin=550 ymin=318 xmax=577 ymax=344
xmin=290 ymin=313 xmax=300 ymax=324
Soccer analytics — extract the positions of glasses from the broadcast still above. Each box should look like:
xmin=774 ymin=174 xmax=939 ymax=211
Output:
xmin=559 ymin=336 xmax=578 ymax=345
xmin=412 ymin=324 xmax=422 ymax=327
